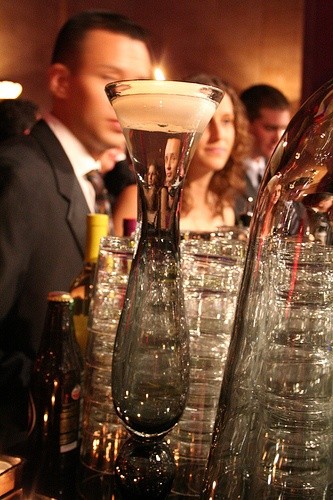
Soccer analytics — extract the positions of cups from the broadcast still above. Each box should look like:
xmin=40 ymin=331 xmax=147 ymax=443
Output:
xmin=250 ymin=238 xmax=333 ymax=499
xmin=75 ymin=227 xmax=251 ymax=498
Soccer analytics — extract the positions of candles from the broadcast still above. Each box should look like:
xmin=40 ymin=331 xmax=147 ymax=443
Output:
xmin=116 ymin=63 xmax=215 ymax=131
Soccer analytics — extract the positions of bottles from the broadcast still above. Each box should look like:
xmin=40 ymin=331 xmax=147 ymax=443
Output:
xmin=28 ymin=289 xmax=84 ymax=474
xmin=120 ymin=217 xmax=138 ymax=240
xmin=198 ymin=76 xmax=333 ymax=500
xmin=99 ymin=77 xmax=227 ymax=499
xmin=67 ymin=213 xmax=111 ymax=355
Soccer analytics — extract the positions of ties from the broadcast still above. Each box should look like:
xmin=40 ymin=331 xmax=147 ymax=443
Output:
xmin=87 ymin=170 xmax=107 ymax=213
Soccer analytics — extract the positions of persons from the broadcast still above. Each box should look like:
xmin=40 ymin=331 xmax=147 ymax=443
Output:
xmin=0 ymin=12 xmax=290 ymax=455
